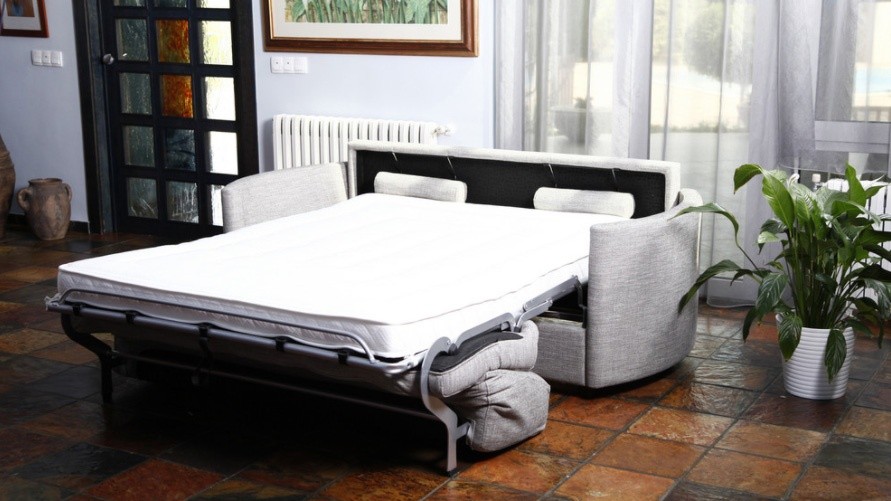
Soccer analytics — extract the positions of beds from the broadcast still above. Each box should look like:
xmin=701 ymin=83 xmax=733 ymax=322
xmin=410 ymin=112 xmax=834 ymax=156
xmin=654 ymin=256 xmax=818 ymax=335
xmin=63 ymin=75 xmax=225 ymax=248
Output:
xmin=42 ymin=144 xmax=703 ymax=475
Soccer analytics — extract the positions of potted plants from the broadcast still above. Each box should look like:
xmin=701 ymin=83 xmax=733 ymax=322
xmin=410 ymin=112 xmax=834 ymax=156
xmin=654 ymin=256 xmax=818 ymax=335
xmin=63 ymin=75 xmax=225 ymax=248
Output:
xmin=549 ymin=98 xmax=609 ymax=144
xmin=662 ymin=164 xmax=891 ymax=400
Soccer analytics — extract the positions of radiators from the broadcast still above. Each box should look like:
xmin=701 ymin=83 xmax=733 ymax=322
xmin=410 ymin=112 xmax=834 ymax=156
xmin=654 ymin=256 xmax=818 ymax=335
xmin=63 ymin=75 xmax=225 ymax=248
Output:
xmin=812 ymin=171 xmax=891 ymax=316
xmin=272 ymin=113 xmax=438 ymax=169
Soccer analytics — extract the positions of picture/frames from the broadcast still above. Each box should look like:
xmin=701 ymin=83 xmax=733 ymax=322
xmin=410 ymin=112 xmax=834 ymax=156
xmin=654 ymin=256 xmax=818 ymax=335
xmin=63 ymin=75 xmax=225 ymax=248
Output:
xmin=0 ymin=0 xmax=50 ymax=39
xmin=261 ymin=0 xmax=478 ymax=57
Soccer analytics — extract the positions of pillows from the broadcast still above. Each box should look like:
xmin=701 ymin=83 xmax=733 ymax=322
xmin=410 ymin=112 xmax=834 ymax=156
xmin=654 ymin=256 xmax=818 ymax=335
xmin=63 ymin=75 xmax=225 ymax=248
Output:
xmin=533 ymin=186 xmax=636 ymax=218
xmin=374 ymin=171 xmax=467 ymax=203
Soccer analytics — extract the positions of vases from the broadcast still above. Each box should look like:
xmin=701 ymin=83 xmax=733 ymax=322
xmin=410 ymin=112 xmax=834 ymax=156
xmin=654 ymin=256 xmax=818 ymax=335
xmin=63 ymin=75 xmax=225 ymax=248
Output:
xmin=17 ymin=178 xmax=73 ymax=240
xmin=0 ymin=136 xmax=16 ymax=239
